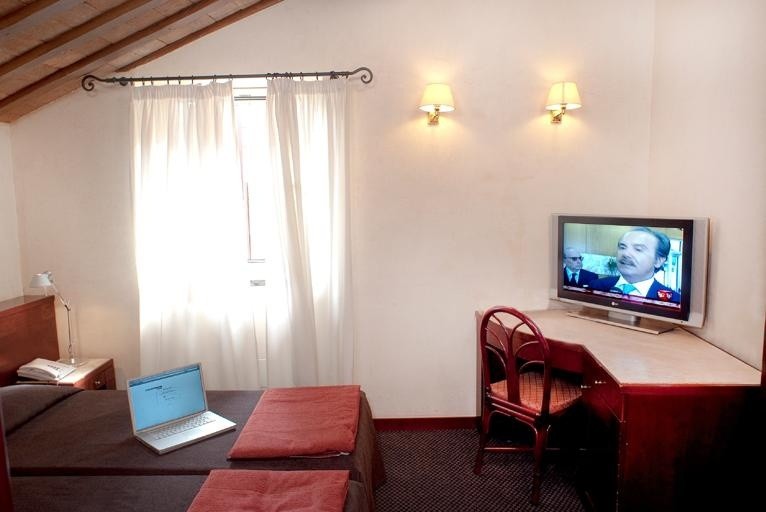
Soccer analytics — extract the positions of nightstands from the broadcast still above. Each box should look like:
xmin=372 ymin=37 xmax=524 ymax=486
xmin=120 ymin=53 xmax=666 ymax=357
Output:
xmin=14 ymin=355 xmax=117 ymax=393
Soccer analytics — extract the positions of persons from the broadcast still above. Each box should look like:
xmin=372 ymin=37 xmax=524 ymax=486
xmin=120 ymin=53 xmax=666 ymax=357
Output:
xmin=563 ymin=248 xmax=598 ymax=289
xmin=591 ymin=227 xmax=681 ymax=304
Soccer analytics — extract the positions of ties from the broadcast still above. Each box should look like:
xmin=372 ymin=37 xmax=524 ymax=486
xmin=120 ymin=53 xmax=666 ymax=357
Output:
xmin=622 ymin=284 xmax=637 ymax=294
xmin=570 ymin=273 xmax=578 ymax=285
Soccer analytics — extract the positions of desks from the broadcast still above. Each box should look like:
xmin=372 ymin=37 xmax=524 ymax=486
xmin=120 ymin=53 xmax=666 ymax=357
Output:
xmin=474 ymin=298 xmax=765 ymax=510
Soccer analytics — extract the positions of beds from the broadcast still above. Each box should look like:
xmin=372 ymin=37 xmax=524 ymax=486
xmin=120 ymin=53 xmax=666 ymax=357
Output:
xmin=9 ymin=469 xmax=373 ymax=512
xmin=0 ymin=293 xmax=387 ymax=508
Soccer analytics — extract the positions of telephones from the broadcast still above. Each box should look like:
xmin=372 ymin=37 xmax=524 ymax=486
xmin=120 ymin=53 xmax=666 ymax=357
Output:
xmin=17 ymin=357 xmax=75 ymax=382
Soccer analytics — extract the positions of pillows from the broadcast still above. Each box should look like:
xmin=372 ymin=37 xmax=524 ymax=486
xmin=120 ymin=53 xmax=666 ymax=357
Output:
xmin=0 ymin=378 xmax=85 ymax=440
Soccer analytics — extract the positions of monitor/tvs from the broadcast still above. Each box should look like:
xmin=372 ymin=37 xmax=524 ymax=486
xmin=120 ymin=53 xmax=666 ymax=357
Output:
xmin=549 ymin=213 xmax=710 ymax=336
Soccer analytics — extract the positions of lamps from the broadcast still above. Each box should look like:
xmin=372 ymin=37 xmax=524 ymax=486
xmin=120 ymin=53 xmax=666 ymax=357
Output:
xmin=26 ymin=268 xmax=87 ymax=371
xmin=542 ymin=80 xmax=583 ymax=126
xmin=415 ymin=82 xmax=455 ymax=128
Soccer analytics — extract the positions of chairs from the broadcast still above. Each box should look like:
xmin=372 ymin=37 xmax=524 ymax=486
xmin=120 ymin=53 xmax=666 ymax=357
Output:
xmin=470 ymin=302 xmax=588 ymax=505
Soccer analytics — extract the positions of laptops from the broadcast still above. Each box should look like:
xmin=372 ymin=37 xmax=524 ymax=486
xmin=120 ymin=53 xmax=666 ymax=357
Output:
xmin=125 ymin=362 xmax=237 ymax=456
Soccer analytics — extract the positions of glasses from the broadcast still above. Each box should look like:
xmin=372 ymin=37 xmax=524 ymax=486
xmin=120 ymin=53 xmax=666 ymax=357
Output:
xmin=567 ymin=256 xmax=584 ymax=262
